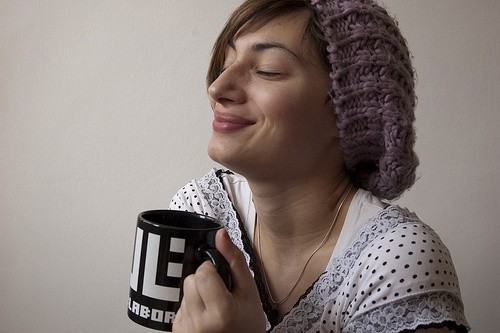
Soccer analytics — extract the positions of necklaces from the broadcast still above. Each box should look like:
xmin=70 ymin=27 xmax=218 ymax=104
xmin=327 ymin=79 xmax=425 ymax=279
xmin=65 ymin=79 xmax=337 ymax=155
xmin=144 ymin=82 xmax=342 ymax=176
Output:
xmin=259 ymin=181 xmax=356 ymax=304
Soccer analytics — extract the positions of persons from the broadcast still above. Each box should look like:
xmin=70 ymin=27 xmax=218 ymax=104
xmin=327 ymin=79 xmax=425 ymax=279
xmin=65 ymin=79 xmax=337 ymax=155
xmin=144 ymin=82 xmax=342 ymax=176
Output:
xmin=164 ymin=0 xmax=472 ymax=333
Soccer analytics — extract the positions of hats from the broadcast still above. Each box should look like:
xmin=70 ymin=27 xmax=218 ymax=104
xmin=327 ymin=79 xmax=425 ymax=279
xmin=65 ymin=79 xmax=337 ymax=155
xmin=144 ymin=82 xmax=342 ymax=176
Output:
xmin=310 ymin=0 xmax=420 ymax=200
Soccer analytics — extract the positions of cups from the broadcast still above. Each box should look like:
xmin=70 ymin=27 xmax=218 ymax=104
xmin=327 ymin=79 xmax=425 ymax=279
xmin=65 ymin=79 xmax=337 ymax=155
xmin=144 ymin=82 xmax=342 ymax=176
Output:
xmin=127 ymin=210 xmax=232 ymax=332
